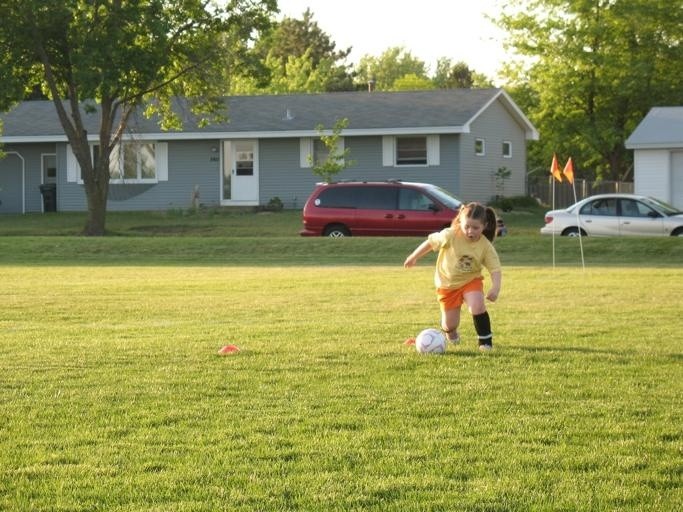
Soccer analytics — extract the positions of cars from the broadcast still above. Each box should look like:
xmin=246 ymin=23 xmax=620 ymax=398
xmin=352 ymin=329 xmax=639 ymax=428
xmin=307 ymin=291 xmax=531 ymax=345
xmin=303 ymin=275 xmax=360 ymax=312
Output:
xmin=539 ymin=193 xmax=683 ymax=237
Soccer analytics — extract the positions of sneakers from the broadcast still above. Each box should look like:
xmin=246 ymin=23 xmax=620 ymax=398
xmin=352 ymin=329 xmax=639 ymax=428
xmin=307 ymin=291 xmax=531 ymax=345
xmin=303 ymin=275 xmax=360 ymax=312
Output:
xmin=478 ymin=342 xmax=493 ymax=350
xmin=448 ymin=336 xmax=460 ymax=345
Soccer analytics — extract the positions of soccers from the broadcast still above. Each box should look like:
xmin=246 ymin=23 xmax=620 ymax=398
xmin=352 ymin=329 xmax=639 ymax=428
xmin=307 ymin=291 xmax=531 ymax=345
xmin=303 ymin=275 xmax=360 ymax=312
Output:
xmin=417 ymin=328 xmax=446 ymax=354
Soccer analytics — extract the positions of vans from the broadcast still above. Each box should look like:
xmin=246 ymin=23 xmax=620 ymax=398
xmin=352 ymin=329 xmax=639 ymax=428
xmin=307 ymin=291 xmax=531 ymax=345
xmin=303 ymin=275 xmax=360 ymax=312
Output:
xmin=300 ymin=179 xmax=509 ymax=238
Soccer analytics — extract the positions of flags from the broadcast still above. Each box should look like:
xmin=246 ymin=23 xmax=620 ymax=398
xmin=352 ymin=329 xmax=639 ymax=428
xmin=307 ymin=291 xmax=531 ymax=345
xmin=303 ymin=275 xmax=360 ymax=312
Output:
xmin=563 ymin=156 xmax=573 ymax=184
xmin=550 ymin=154 xmax=562 ymax=183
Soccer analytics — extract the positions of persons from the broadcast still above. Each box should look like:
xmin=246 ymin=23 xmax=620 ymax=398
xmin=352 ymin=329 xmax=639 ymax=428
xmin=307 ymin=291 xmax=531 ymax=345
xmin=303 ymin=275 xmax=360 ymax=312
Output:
xmin=404 ymin=202 xmax=502 ymax=350
xmin=497 ymin=218 xmax=507 ymax=236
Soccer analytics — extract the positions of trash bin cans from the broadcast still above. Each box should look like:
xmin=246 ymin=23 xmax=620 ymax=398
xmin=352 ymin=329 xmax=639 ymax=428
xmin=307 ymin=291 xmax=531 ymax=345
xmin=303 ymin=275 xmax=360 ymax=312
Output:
xmin=40 ymin=184 xmax=56 ymax=212
